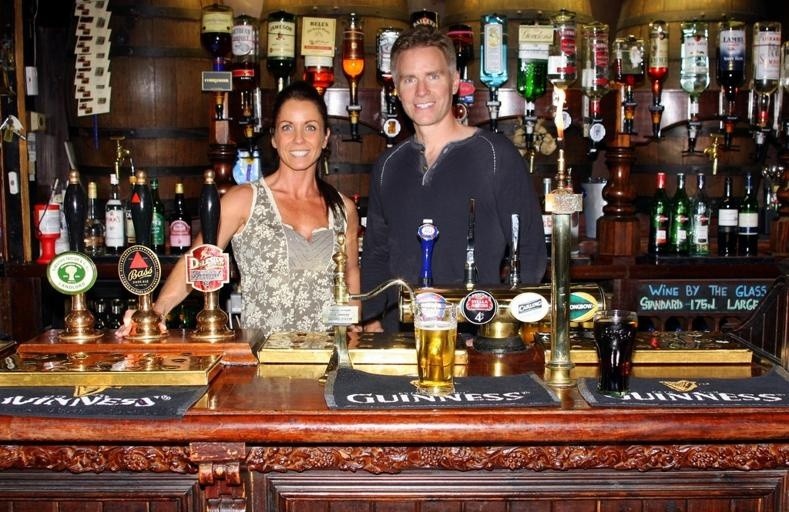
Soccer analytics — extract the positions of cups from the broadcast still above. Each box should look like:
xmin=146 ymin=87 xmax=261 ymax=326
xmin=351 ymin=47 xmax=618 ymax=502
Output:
xmin=414 ymin=299 xmax=461 ymax=390
xmin=593 ymin=310 xmax=638 ymax=392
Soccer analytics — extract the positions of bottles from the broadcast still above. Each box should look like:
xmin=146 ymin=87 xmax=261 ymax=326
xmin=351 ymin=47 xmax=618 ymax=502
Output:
xmin=612 ymin=35 xmax=646 ymax=136
xmin=108 ymin=299 xmax=120 ymax=328
xmin=669 ymin=172 xmax=689 ymax=254
xmin=342 ymin=15 xmax=365 ymax=143
xmin=649 ymin=170 xmax=668 ymax=257
xmin=447 ymin=24 xmax=474 ymax=124
xmin=84 ymin=182 xmax=104 ymax=255
xmin=63 ymin=164 xmax=86 ymax=253
xmin=680 ymin=19 xmax=710 ymax=153
xmin=376 ymin=27 xmax=404 ymax=148
xmin=581 ymin=21 xmax=611 ymax=160
xmin=148 ymin=177 xmax=165 ymax=255
xmin=408 ymin=7 xmax=439 ymax=31
xmin=718 ymin=177 xmax=739 ymax=258
xmin=202 ymin=3 xmax=233 ymax=120
xmin=169 ymin=182 xmax=192 ymax=255
xmin=105 ymin=174 xmax=124 ymax=255
xmin=647 ymin=20 xmax=669 ymax=140
xmin=738 ymin=173 xmax=760 ymax=257
xmin=715 ymin=14 xmax=745 ymax=151
xmin=124 ymin=176 xmax=137 ymax=253
xmin=231 ymin=14 xmax=259 ymax=156
xmin=690 ymin=173 xmax=711 ymax=255
xmin=547 ymin=21 xmax=578 ymax=145
xmin=516 ymin=21 xmax=549 ymax=152
xmin=752 ymin=21 xmax=780 ymax=160
xmin=266 ymin=11 xmax=297 ymax=92
xmin=94 ymin=299 xmax=106 ymax=329
xmin=478 ymin=13 xmax=509 ymax=133
xmin=301 ymin=17 xmax=338 ymax=174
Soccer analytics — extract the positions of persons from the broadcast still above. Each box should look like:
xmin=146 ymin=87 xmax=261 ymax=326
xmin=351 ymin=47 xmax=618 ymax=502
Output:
xmin=154 ymin=80 xmax=361 ymax=337
xmin=360 ymin=24 xmax=549 ymax=333
